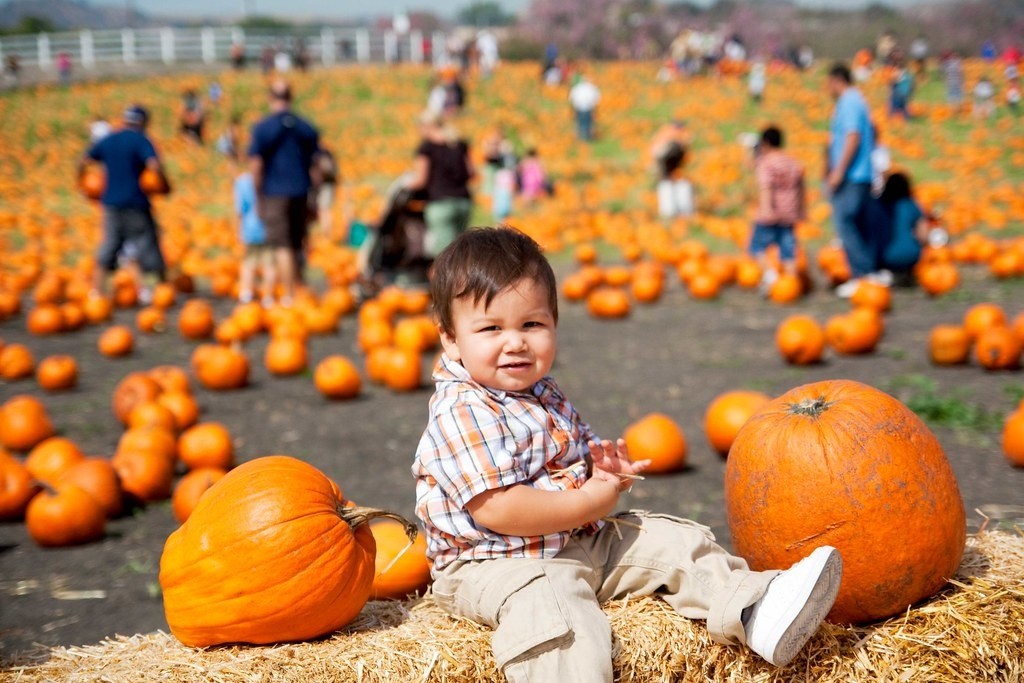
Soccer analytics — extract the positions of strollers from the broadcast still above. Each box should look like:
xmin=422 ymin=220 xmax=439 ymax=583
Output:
xmin=365 ymin=182 xmax=434 ymax=287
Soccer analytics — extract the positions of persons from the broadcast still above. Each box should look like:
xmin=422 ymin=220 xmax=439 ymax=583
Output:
xmin=747 ymin=125 xmax=806 ymax=298
xmin=482 ymin=137 xmax=555 ymax=222
xmin=55 ymin=51 xmax=70 ymax=77
xmin=228 ymin=38 xmax=314 ymax=71
xmin=821 ymin=61 xmax=924 ymax=292
xmin=179 ymin=86 xmax=204 ymax=144
xmin=348 ymin=79 xmax=477 ymax=299
xmin=216 ymin=73 xmax=338 ymax=304
xmin=76 ymin=101 xmax=169 ymax=300
xmin=671 ymin=28 xmax=1024 ymax=116
xmin=653 ymin=134 xmax=693 ymax=218
xmin=410 ymin=224 xmax=843 ymax=683
xmin=568 ymin=74 xmax=602 ymax=142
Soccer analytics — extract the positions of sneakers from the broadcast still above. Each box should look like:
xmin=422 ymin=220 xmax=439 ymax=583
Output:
xmin=744 ymin=545 xmax=843 ymax=668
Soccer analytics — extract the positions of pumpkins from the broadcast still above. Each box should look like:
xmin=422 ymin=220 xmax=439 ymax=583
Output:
xmin=0 ymin=54 xmax=1024 ymax=548
xmin=724 ymin=378 xmax=965 ymax=628
xmin=160 ymin=457 xmax=431 ymax=648
